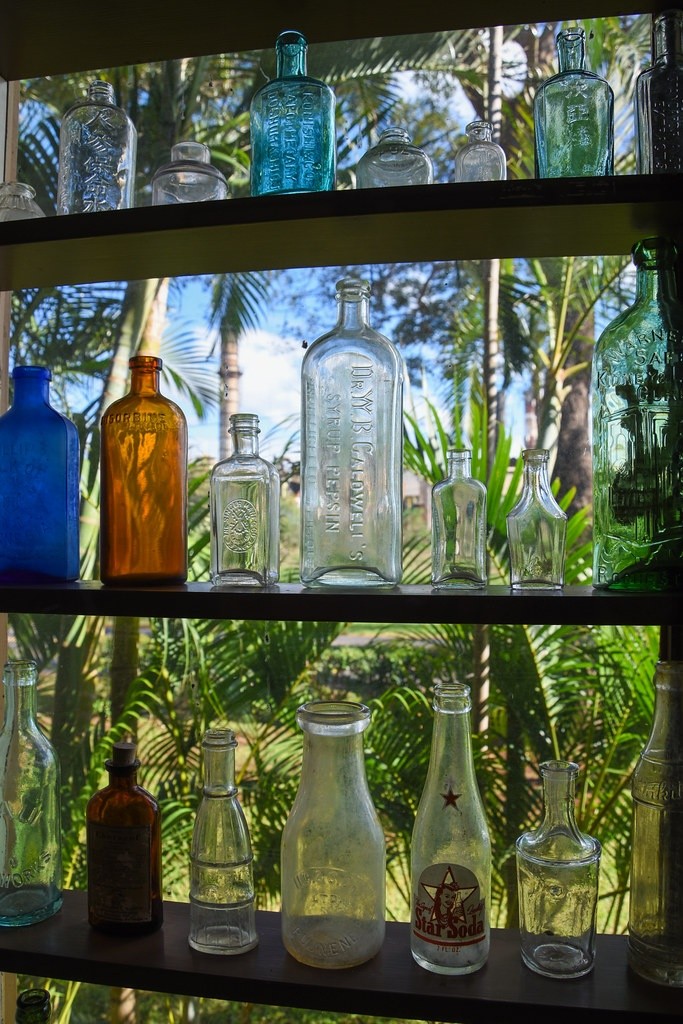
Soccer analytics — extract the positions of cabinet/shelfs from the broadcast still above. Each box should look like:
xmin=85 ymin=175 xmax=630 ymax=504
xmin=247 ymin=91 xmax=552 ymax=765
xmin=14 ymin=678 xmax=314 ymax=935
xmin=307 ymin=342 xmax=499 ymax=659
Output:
xmin=0 ymin=0 xmax=683 ymax=1024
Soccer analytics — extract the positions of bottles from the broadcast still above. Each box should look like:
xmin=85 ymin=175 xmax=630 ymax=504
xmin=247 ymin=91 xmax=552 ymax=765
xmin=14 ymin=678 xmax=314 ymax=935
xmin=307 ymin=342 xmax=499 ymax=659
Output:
xmin=515 ymin=760 xmax=603 ymax=981
xmin=0 ymin=659 xmax=61 ymax=929
xmin=355 ymin=127 xmax=434 ymax=188
xmin=590 ymin=234 xmax=683 ymax=595
xmin=299 ymin=277 xmax=404 ymax=593
xmin=505 ymin=448 xmax=566 ymax=592
xmin=636 ymin=10 xmax=682 ymax=174
xmin=1 ymin=366 xmax=80 ymax=588
xmin=533 ymin=26 xmax=616 ymax=180
xmin=454 ymin=121 xmax=507 ymax=183
xmin=249 ymin=30 xmax=338 ymax=198
xmin=188 ymin=726 xmax=260 ymax=955
xmin=409 ymin=683 xmax=495 ymax=976
xmin=99 ymin=355 xmax=188 ymax=587
xmin=278 ymin=700 xmax=387 ymax=970
xmin=0 ymin=78 xmax=229 ymax=223
xmin=627 ymin=660 xmax=683 ymax=990
xmin=431 ymin=450 xmax=488 ymax=592
xmin=209 ymin=412 xmax=279 ymax=588
xmin=84 ymin=742 xmax=164 ymax=941
